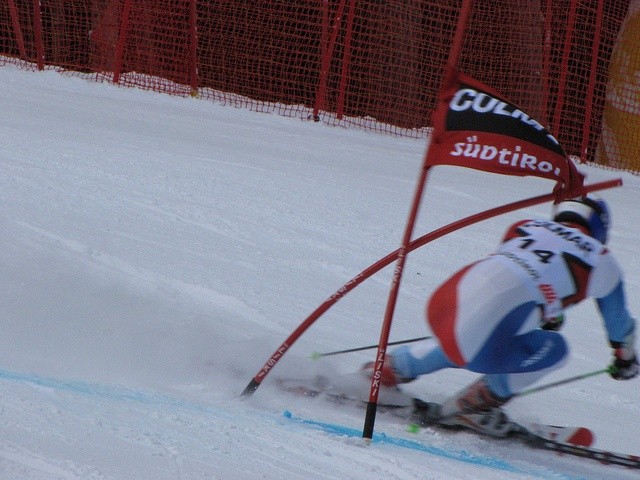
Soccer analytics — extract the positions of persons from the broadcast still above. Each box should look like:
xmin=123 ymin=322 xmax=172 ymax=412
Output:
xmin=355 ymin=191 xmax=640 ymax=438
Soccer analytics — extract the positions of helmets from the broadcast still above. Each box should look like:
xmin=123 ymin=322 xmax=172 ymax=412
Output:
xmin=551 ymin=194 xmax=610 ymax=244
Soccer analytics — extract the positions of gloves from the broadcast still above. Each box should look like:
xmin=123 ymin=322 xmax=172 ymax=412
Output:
xmin=608 ymin=346 xmax=639 ymax=379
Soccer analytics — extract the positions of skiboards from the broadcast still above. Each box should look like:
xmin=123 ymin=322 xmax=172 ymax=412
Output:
xmin=275 ymin=378 xmax=639 ymax=479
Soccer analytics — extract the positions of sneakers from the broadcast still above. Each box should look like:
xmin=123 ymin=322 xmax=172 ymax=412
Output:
xmin=346 ymin=354 xmax=417 ymax=402
xmin=440 ymin=379 xmax=512 ymax=439
